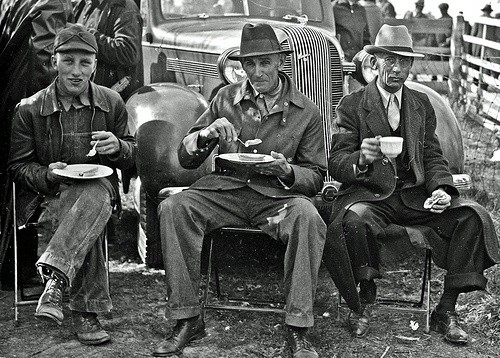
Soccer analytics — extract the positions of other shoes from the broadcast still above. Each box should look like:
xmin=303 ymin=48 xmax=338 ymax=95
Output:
xmin=72 ymin=310 xmax=110 ymax=344
xmin=35 ymin=276 xmax=63 ymax=325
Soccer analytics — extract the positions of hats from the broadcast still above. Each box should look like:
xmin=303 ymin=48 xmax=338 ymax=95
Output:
xmin=228 ymin=23 xmax=294 ymax=61
xmin=54 ymin=23 xmax=98 ymax=57
xmin=363 ymin=23 xmax=424 ymax=57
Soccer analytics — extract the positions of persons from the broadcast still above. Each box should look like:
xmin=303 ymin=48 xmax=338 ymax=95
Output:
xmin=0 ymin=0 xmax=142 ymax=345
xmin=324 ymin=23 xmax=500 ymax=343
xmin=331 ymin=0 xmax=500 ymax=91
xmin=152 ymin=23 xmax=330 ymax=358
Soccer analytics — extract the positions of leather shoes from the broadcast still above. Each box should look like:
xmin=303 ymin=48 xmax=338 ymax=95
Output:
xmin=153 ymin=316 xmax=206 ymax=357
xmin=287 ymin=326 xmax=318 ymax=358
xmin=429 ymin=306 xmax=468 ymax=341
xmin=349 ymin=293 xmax=374 ymax=338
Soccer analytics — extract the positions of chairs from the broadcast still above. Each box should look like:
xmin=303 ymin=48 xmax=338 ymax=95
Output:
xmin=202 ymin=226 xmax=285 ymax=316
xmin=0 ymin=181 xmax=113 ymax=326
xmin=337 ymin=229 xmax=431 ymax=335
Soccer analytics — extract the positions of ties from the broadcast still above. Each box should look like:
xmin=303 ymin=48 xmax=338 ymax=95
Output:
xmin=387 ymin=95 xmax=400 ymax=130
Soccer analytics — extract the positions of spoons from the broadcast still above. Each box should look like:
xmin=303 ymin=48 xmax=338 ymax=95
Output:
xmin=237 ymin=138 xmax=262 ymax=146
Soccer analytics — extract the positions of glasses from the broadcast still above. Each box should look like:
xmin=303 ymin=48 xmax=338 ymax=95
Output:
xmin=376 ymin=58 xmax=412 ymax=67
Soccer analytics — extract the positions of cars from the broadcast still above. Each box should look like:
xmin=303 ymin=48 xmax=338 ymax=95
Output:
xmin=124 ymin=0 xmax=474 ymax=270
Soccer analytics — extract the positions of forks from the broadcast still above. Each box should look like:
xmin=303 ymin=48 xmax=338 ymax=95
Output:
xmin=86 ymin=130 xmax=105 ymax=157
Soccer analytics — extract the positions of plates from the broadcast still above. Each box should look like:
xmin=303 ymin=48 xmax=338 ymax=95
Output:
xmin=53 ymin=164 xmax=113 ymax=179
xmin=219 ymin=153 xmax=276 ymax=164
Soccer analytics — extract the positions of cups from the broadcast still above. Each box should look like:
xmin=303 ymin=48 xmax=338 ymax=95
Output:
xmin=376 ymin=136 xmax=403 ymax=158
